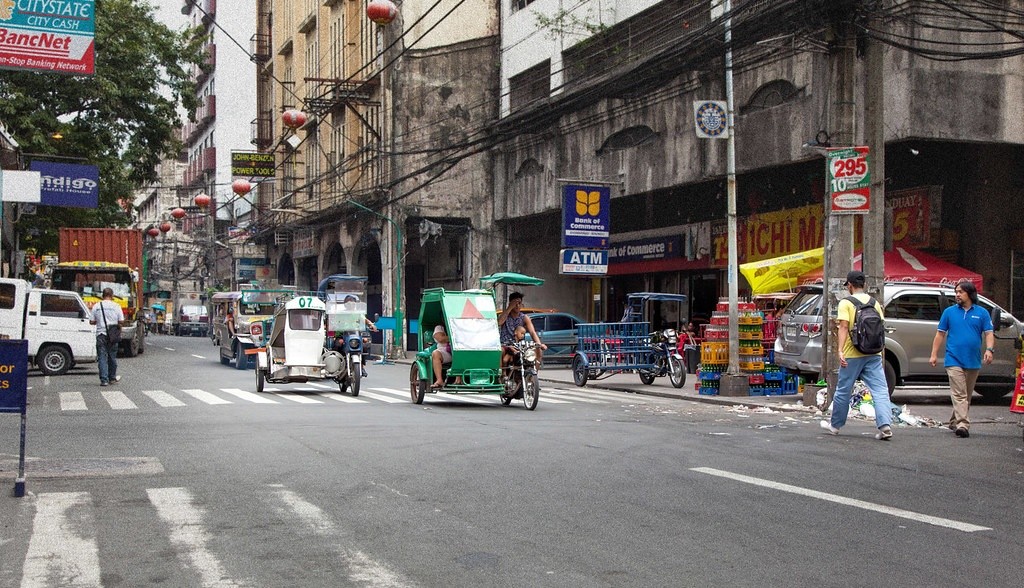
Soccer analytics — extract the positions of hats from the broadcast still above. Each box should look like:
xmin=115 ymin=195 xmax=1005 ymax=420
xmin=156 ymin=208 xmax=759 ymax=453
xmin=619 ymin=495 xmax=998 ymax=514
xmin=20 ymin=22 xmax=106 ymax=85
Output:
xmin=843 ymin=270 xmax=866 ymax=286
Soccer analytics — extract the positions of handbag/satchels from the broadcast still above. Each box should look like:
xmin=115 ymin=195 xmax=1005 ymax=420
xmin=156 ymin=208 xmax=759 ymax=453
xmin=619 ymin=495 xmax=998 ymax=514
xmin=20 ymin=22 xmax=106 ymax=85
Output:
xmin=107 ymin=324 xmax=122 ymax=343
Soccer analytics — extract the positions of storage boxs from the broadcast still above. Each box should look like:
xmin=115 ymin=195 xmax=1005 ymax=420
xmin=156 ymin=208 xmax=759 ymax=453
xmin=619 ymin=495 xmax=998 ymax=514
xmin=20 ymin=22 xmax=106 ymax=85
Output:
xmin=696 ymin=303 xmax=800 ymax=397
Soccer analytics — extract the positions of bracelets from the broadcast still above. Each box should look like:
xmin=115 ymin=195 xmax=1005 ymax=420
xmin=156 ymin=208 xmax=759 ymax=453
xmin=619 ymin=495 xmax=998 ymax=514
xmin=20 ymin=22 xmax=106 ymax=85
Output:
xmin=685 ymin=330 xmax=688 ymax=332
xmin=984 ymin=347 xmax=995 ymax=354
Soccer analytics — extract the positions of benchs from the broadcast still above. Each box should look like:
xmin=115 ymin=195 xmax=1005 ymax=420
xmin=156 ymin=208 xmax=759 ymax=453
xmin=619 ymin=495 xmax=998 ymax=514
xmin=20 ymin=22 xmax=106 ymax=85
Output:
xmin=272 ymin=346 xmax=285 ymax=363
xmin=425 ymin=329 xmax=452 ymax=385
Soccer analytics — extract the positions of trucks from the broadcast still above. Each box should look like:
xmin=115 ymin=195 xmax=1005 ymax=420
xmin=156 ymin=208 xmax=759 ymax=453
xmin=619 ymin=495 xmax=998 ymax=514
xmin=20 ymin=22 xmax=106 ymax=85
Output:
xmin=173 ymin=299 xmax=209 ymax=337
xmin=0 ymin=277 xmax=100 ymax=375
xmin=51 ymin=227 xmax=148 ymax=357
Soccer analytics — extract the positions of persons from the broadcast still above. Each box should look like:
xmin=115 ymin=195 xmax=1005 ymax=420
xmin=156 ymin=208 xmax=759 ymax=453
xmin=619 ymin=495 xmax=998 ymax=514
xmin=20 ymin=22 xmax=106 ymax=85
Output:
xmin=774 ymin=304 xmax=786 ymax=338
xmin=927 ymin=282 xmax=996 ymax=438
xmin=819 ymin=271 xmax=895 ymax=439
xmin=429 ymin=310 xmax=463 ymax=389
xmin=620 ymin=302 xmax=636 ymax=322
xmin=89 ymin=287 xmax=121 ymax=387
xmin=144 ymin=309 xmax=165 ymax=335
xmin=681 ymin=322 xmax=696 ymax=338
xmin=498 ymin=292 xmax=548 ymax=379
xmin=224 ymin=306 xmax=236 ymax=337
xmin=765 ymin=303 xmax=774 ymax=349
xmin=331 ymin=295 xmax=378 ymax=376
xmin=503 ymin=325 xmax=528 ymax=378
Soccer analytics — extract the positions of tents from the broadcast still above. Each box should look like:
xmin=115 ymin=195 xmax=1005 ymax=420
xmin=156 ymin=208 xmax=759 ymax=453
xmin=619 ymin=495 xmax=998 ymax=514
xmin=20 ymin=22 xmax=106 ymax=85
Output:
xmin=796 ymin=246 xmax=984 ymax=294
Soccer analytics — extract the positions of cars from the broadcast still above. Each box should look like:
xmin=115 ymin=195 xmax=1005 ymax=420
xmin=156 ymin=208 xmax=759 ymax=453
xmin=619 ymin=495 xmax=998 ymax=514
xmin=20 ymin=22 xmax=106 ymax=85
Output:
xmin=210 ymin=287 xmax=285 ymax=369
xmin=497 ymin=308 xmax=615 ymax=363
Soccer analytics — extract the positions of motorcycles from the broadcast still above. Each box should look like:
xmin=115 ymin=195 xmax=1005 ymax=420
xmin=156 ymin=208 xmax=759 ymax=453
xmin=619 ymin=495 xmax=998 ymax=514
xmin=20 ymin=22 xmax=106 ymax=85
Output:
xmin=255 ymin=274 xmax=381 ymax=397
xmin=409 ymin=272 xmax=546 ymax=410
xmin=571 ymin=321 xmax=687 ymax=388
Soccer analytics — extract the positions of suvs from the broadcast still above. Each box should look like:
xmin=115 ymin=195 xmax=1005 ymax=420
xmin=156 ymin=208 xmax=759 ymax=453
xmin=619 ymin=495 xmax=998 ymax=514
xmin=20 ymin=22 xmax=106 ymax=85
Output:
xmin=772 ymin=281 xmax=1024 ymax=402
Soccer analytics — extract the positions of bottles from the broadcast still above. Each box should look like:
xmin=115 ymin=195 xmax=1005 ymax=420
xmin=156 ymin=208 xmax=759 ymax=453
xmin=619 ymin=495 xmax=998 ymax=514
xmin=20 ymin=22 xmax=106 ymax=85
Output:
xmin=695 ymin=296 xmax=806 ymax=397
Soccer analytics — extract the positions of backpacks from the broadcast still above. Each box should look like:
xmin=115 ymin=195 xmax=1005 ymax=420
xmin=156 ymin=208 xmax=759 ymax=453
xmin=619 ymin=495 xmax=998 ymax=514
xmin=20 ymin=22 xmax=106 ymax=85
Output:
xmin=842 ymin=295 xmax=887 ymax=355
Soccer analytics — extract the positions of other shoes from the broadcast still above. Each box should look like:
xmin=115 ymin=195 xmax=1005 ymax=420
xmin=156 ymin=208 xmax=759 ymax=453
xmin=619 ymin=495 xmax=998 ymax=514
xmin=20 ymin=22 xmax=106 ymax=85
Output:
xmin=956 ymin=428 xmax=969 ymax=438
xmin=109 ymin=375 xmax=122 ymax=385
xmin=429 ymin=381 xmax=444 ymax=388
xmin=820 ymin=420 xmax=839 ymax=435
xmin=100 ymin=382 xmax=108 ymax=386
xmin=949 ymin=422 xmax=957 ymax=432
xmin=875 ymin=426 xmax=893 ymax=440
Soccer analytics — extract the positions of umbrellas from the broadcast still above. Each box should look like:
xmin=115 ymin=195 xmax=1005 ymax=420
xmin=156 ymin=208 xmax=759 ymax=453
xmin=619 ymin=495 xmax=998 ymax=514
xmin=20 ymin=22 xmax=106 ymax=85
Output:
xmin=151 ymin=304 xmax=166 ymax=311
xmin=740 ymin=248 xmax=824 ymax=297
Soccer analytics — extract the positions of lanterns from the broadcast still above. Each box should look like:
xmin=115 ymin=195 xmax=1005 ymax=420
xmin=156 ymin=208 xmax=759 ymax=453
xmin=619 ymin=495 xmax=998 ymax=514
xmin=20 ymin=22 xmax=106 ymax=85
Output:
xmin=232 ymin=179 xmax=251 ymax=197
xmin=283 ymin=110 xmax=307 ymax=130
xmin=195 ymin=192 xmax=211 ymax=211
xmin=148 ymin=227 xmax=158 ymax=238
xmin=172 ymin=208 xmax=185 ymax=219
xmin=158 ymin=222 xmax=170 ymax=234
xmin=367 ymin=0 xmax=398 ymax=26
